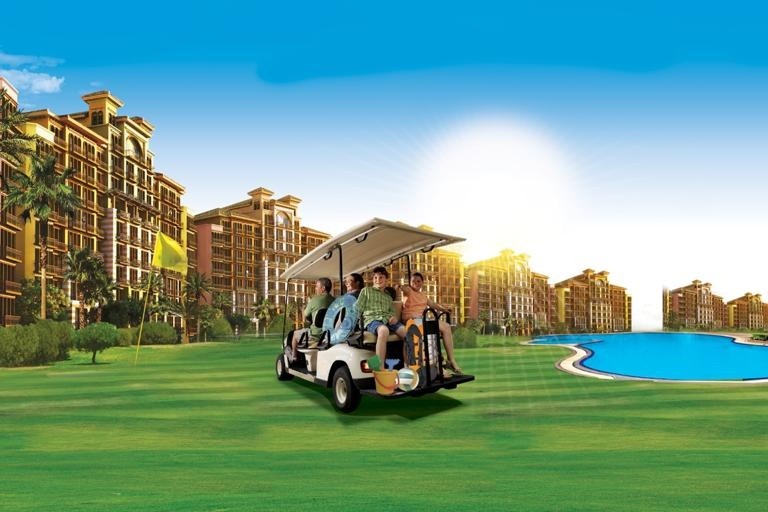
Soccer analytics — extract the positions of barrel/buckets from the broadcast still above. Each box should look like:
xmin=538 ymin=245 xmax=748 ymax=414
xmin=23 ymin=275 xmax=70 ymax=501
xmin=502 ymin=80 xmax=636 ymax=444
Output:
xmin=372 ymin=369 xmax=400 ymax=396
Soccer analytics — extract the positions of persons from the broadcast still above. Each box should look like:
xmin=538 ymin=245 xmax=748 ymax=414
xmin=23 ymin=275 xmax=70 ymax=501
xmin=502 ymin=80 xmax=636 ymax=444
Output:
xmin=394 ymin=273 xmax=464 ymax=379
xmin=323 ymin=273 xmax=363 ymax=344
xmin=384 ymin=287 xmax=397 ymax=302
xmin=289 ymin=277 xmax=336 ymax=363
xmin=358 ymin=267 xmax=409 ymax=370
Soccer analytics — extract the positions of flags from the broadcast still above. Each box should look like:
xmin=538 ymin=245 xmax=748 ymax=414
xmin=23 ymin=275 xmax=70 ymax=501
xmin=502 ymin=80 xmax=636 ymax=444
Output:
xmin=151 ymin=231 xmax=189 ymax=276
